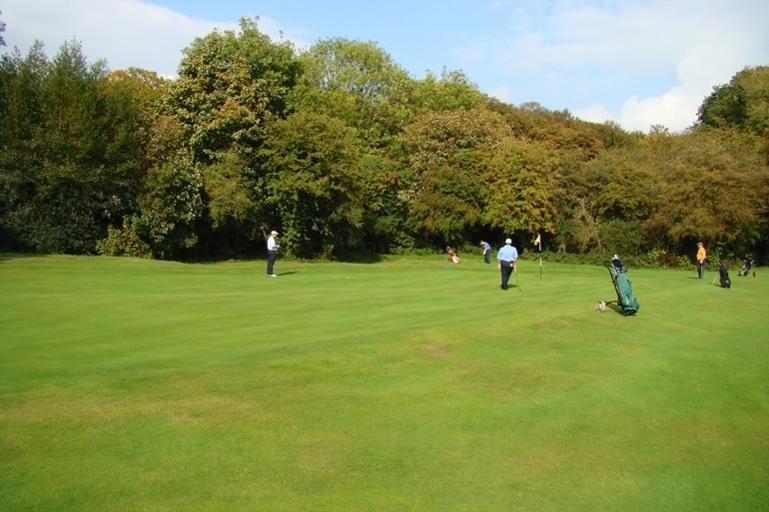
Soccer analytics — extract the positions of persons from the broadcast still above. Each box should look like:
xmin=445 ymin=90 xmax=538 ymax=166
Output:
xmin=611 ymin=254 xmax=623 ymax=273
xmin=480 ymin=239 xmax=492 ymax=264
xmin=696 ymin=242 xmax=707 ymax=280
xmin=497 ymin=238 xmax=518 ymax=290
xmin=265 ymin=230 xmax=280 ymax=278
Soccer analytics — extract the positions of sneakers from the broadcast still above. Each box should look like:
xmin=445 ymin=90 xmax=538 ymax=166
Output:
xmin=266 ymin=273 xmax=278 ymax=278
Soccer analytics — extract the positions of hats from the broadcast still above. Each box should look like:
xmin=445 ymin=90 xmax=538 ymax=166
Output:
xmin=505 ymin=238 xmax=512 ymax=244
xmin=271 ymin=231 xmax=279 ymax=235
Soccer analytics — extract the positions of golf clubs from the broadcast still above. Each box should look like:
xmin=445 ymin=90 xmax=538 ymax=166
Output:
xmin=514 ymin=262 xmax=522 ymax=292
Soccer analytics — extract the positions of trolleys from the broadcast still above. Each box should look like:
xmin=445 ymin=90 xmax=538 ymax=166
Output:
xmin=711 ymin=253 xmax=731 ymax=288
xmin=595 ymin=254 xmax=639 ymax=316
xmin=739 ymin=253 xmax=757 ymax=277
